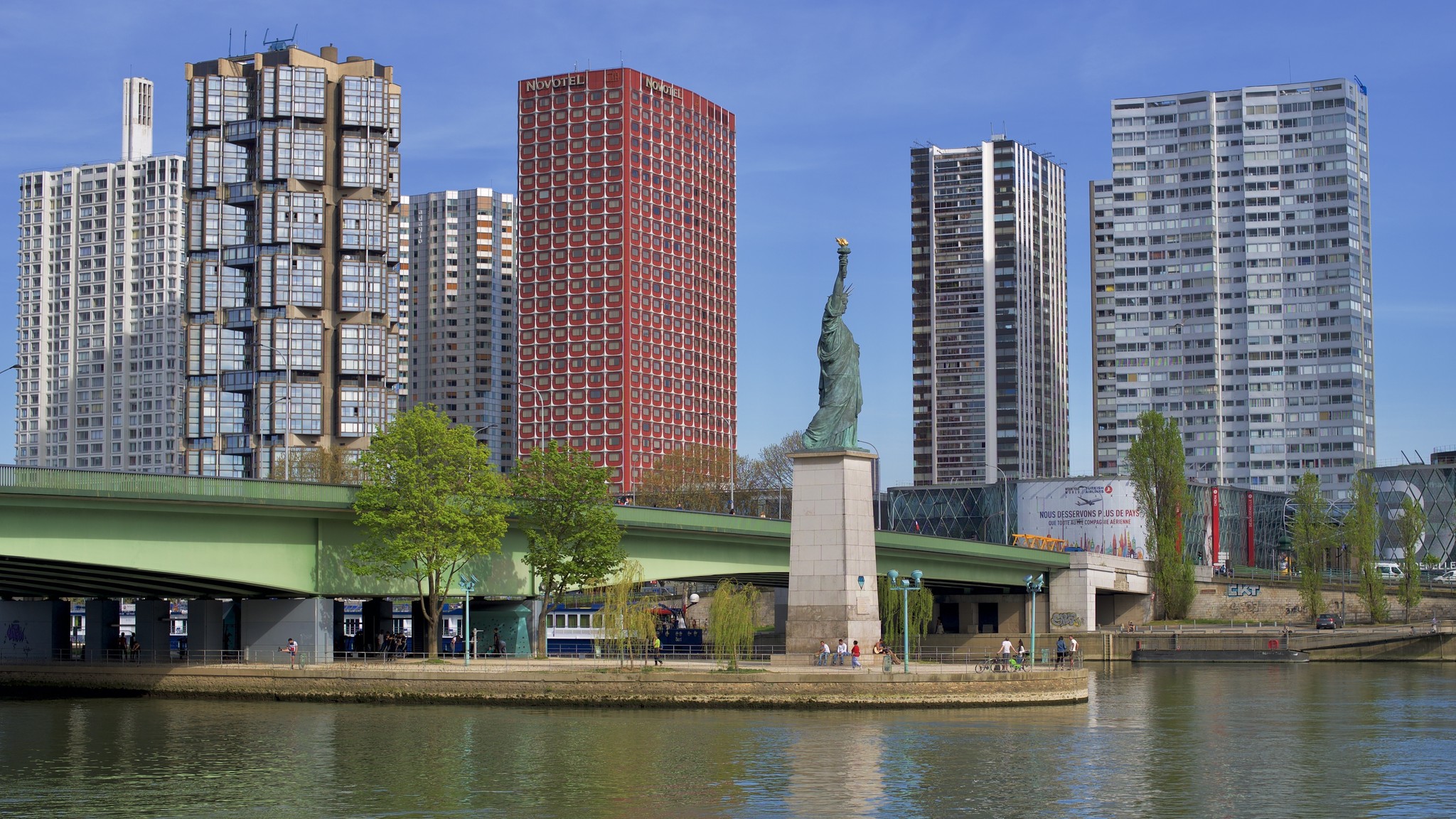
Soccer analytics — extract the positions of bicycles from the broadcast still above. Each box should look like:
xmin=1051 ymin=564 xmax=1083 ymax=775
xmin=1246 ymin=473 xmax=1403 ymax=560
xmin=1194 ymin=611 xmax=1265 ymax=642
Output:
xmin=975 ymin=653 xmax=1004 ymax=673
xmin=1007 ymin=650 xmax=1031 ymax=673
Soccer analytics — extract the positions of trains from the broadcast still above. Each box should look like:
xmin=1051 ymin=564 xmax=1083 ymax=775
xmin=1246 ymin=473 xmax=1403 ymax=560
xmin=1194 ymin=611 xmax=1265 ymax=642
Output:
xmin=70 ymin=604 xmax=703 ymax=662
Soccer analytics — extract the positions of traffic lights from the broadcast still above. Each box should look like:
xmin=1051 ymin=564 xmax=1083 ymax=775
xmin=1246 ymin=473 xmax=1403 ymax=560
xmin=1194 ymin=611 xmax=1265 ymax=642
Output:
xmin=1198 ymin=551 xmax=1202 ymax=560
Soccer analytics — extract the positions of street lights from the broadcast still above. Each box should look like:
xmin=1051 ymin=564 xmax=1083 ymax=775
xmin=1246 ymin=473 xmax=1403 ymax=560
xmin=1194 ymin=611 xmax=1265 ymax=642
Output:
xmin=887 ymin=491 xmax=916 ymax=531
xmin=887 ymin=569 xmax=923 ymax=673
xmin=1220 ymin=506 xmax=1231 ymax=572
xmin=1268 ymin=549 xmax=1274 ymax=582
xmin=1078 ymin=485 xmax=1104 ymax=554
xmin=509 ymin=382 xmax=544 ymax=491
xmin=701 ymin=412 xmax=733 ymax=511
xmin=457 ymin=573 xmax=480 ymax=665
xmin=1022 ymin=572 xmax=1044 ymax=666
xmin=633 ymin=447 xmax=662 ymax=506
xmin=984 ymin=511 xmax=1005 ymax=542
xmin=468 ymin=424 xmax=498 ymax=483
xmin=249 ymin=342 xmax=289 ymax=482
xmin=260 ymin=396 xmax=291 ymax=478
xmin=979 ymin=463 xmax=1008 ymax=545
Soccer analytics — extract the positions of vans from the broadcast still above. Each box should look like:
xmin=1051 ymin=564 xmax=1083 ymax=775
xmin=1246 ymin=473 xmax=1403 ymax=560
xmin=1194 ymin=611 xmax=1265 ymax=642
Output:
xmin=1361 ymin=561 xmax=1406 ymax=581
xmin=1419 ymin=570 xmax=1447 ymax=582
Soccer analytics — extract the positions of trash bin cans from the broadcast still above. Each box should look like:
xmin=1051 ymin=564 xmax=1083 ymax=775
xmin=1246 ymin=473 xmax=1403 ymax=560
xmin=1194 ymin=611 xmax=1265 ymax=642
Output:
xmin=882 ymin=656 xmax=892 ymax=674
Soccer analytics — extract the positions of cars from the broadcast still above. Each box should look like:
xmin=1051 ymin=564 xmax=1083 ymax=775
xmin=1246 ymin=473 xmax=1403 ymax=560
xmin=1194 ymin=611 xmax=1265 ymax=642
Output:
xmin=1433 ymin=570 xmax=1456 ymax=584
xmin=1316 ymin=613 xmax=1343 ymax=630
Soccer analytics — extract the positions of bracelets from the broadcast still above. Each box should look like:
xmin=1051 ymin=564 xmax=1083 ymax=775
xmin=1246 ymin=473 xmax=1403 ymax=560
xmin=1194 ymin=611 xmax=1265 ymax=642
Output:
xmin=1072 ymin=649 xmax=1073 ymax=650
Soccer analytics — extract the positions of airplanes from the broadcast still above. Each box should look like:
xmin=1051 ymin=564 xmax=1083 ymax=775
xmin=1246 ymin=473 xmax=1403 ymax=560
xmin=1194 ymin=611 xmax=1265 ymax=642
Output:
xmin=1075 ymin=496 xmax=1103 ymax=506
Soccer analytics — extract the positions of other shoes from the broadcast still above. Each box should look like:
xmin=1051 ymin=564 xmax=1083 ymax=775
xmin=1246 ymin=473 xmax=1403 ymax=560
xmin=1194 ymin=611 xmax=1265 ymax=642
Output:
xmin=292 ymin=665 xmax=294 ymax=669
xmin=817 ymin=662 xmax=822 ymax=665
xmin=654 ymin=665 xmax=658 ymax=666
xmin=851 ymin=668 xmax=856 ymax=670
xmin=278 ymin=647 xmax=281 ymax=652
xmin=860 ymin=665 xmax=862 ymax=669
xmin=500 ymin=656 xmax=503 ymax=657
xmin=660 ymin=662 xmax=663 ymax=666
xmin=841 ymin=662 xmax=843 ymax=665
xmin=832 ymin=662 xmax=836 ymax=665
xmin=824 ymin=662 xmax=826 ymax=666
xmin=452 ymin=657 xmax=456 ymax=659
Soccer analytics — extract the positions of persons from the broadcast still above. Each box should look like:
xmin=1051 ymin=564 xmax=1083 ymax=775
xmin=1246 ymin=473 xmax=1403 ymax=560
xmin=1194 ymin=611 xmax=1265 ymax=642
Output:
xmin=729 ymin=509 xmax=735 ymax=514
xmin=278 ymin=638 xmax=298 ymax=669
xmin=997 ymin=637 xmax=1024 ymax=672
xmin=353 ymin=631 xmax=364 ymax=656
xmin=671 ymin=618 xmax=679 ymax=629
xmin=653 ymin=634 xmax=663 ymax=666
xmin=1220 ymin=565 xmax=1225 ymax=575
xmin=876 ymin=639 xmax=888 ymax=653
xmin=387 ymin=597 xmax=390 ymax=601
xmin=451 ymin=628 xmax=506 ymax=659
xmin=119 ymin=632 xmax=141 ymax=666
xmin=132 ymin=599 xmax=134 ymax=603
xmin=654 ymin=503 xmax=659 ymax=507
xmin=760 ymin=511 xmax=765 ymax=518
xmin=617 ymin=497 xmax=634 ymax=506
xmin=1427 ymin=624 xmax=1436 ymax=633
xmin=702 ymin=619 xmax=709 ymax=636
xmin=676 ymin=503 xmax=683 ymax=510
xmin=1127 ymin=622 xmax=1135 ymax=634
xmin=377 ymin=629 xmax=408 ymax=662
xmin=816 ymin=639 xmax=862 ymax=670
xmin=1281 ymin=624 xmax=1289 ymax=633
xmin=1053 ymin=635 xmax=1078 ymax=671
xmin=803 ymin=255 xmax=862 ymax=449
xmin=1407 ymin=627 xmax=1416 ymax=636
